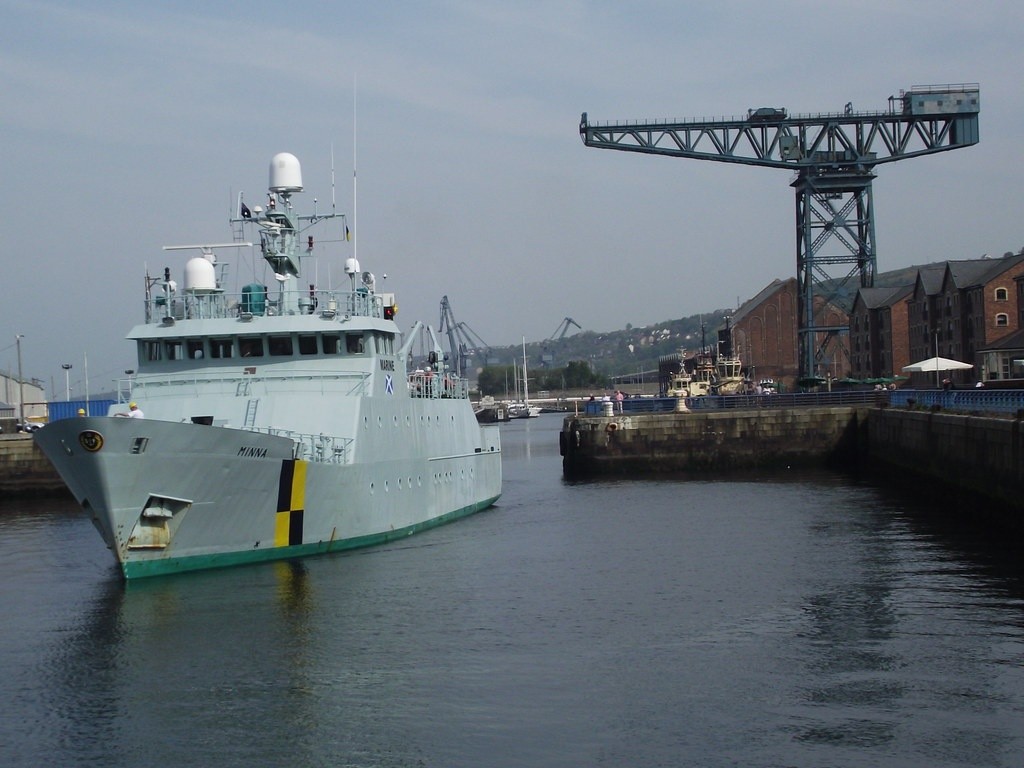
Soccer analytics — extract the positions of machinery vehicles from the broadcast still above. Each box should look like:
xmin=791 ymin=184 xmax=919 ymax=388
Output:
xmin=539 ymin=317 xmax=582 ymax=363
xmin=438 ymin=295 xmax=487 ymax=369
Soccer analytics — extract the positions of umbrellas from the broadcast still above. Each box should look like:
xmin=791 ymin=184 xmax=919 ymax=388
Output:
xmin=901 ymin=356 xmax=973 ymax=387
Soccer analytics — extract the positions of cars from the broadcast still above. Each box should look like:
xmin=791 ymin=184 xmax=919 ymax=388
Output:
xmin=0 ymin=419 xmax=45 ymax=433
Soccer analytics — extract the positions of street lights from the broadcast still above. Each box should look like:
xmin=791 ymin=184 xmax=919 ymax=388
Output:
xmin=61 ymin=364 xmax=73 ymax=401
xmin=125 ymin=370 xmax=134 ymax=393
xmin=931 ymin=328 xmax=940 ymax=388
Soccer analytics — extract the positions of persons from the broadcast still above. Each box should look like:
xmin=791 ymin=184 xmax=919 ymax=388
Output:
xmin=975 ymin=380 xmax=984 ymax=387
xmin=754 ymin=382 xmax=762 ymax=408
xmin=422 ymin=366 xmax=433 ymax=384
xmin=114 ymin=402 xmax=144 ymax=419
xmin=601 ymin=392 xmax=609 ymax=401
xmin=943 ymin=377 xmax=950 ymax=383
xmin=588 ymin=393 xmax=595 ymax=402
xmin=692 ymin=369 xmax=698 ymax=382
xmin=614 ymin=390 xmax=624 ymax=414
xmin=78 ymin=408 xmax=86 ymax=418
xmin=668 ymin=372 xmax=675 ymax=389
xmin=710 ymin=373 xmax=717 ymax=393
xmin=873 ymin=383 xmax=896 ymax=391
xmin=760 ymin=378 xmax=775 ymax=393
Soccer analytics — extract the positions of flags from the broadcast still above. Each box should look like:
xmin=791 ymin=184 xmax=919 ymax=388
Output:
xmin=346 ymin=226 xmax=351 ymax=241
xmin=241 ymin=202 xmax=251 ymax=218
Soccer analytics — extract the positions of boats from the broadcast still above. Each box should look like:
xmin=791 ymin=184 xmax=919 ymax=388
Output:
xmin=471 ymin=337 xmax=543 ymax=424
xmin=33 ymin=51 xmax=502 ymax=584
